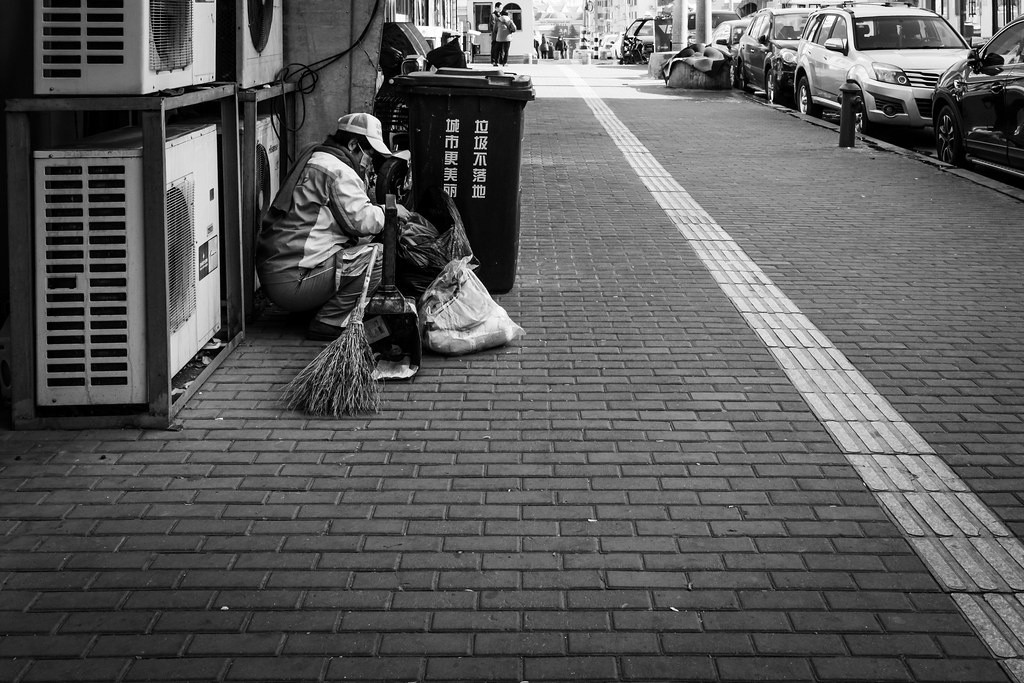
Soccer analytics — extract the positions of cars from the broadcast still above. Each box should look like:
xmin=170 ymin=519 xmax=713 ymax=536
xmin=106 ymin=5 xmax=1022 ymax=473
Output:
xmin=711 ymin=20 xmax=751 ymax=69
xmin=598 ymin=10 xmax=743 ymax=67
xmin=932 ymin=13 xmax=1024 ymax=182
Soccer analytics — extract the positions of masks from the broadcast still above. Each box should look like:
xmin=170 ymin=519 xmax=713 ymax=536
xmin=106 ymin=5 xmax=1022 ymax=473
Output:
xmin=356 ymin=142 xmax=373 ymax=173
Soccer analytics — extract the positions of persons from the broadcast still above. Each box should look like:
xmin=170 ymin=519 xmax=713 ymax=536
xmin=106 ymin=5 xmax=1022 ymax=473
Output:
xmin=493 ymin=10 xmax=517 ymax=66
xmin=489 ymin=1 xmax=504 ymax=65
xmin=548 ymin=42 xmax=555 ymax=58
xmin=555 ymin=36 xmax=567 ymax=59
xmin=254 ymin=113 xmax=412 ymax=342
xmin=535 ymin=33 xmax=549 ymax=58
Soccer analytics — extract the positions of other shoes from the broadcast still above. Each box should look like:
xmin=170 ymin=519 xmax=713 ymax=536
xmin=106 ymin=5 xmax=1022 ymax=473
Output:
xmin=306 ymin=320 xmax=346 ymax=342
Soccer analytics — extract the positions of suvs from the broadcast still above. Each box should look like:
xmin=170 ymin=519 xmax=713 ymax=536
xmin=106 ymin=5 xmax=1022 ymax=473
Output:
xmin=744 ymin=8 xmax=830 ymax=105
xmin=794 ymin=1 xmax=976 ymax=136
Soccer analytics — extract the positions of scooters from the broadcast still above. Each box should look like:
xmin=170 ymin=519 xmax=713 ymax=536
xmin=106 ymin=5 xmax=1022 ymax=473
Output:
xmin=373 ymin=40 xmax=440 ymax=214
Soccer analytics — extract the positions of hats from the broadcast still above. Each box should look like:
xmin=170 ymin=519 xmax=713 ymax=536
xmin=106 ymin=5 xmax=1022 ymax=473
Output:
xmin=337 ymin=112 xmax=393 ymax=156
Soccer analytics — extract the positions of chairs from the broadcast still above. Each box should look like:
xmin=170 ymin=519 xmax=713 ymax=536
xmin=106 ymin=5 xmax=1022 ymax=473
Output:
xmin=855 ymin=25 xmax=873 ymax=48
xmin=900 ymin=21 xmax=924 ymax=46
xmin=878 ymin=21 xmax=900 ymax=42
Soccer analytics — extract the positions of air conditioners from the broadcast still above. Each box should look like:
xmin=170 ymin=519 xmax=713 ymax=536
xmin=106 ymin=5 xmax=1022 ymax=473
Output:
xmin=216 ymin=0 xmax=284 ymax=91
xmin=33 ymin=124 xmax=222 ymax=404
xmin=215 ymin=113 xmax=280 ymax=291
xmin=33 ymin=0 xmax=217 ymax=96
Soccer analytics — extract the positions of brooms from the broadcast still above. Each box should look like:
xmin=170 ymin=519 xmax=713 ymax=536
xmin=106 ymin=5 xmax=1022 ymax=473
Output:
xmin=280 ymin=243 xmax=384 ymax=420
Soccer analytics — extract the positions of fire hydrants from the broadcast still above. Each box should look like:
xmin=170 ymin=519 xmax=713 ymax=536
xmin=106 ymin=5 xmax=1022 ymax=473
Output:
xmin=837 ymin=77 xmax=864 ymax=147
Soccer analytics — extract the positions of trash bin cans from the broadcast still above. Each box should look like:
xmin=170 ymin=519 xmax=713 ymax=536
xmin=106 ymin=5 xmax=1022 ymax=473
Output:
xmin=386 ymin=67 xmax=537 ymax=295
